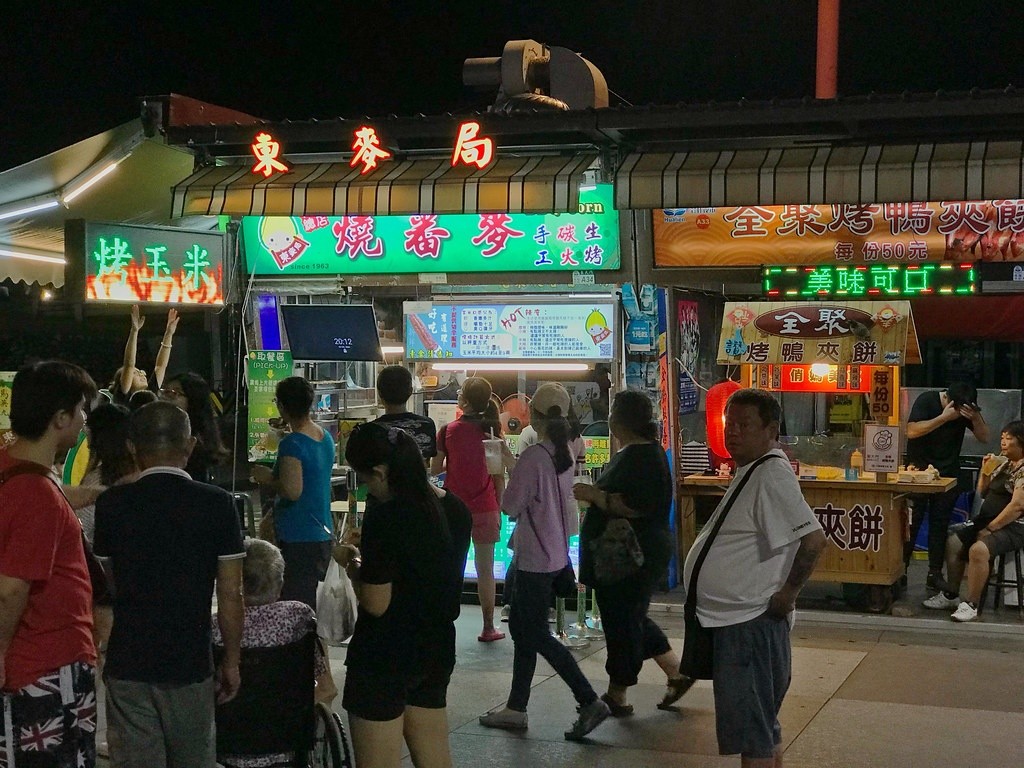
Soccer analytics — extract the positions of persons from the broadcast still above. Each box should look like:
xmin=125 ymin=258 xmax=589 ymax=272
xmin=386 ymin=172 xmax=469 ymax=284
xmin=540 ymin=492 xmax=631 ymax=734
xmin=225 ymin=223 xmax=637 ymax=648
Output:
xmin=572 ymin=390 xmax=697 ymax=717
xmin=683 ymin=388 xmax=826 ymax=768
xmin=478 ymin=384 xmax=609 ymax=740
xmin=903 ymin=382 xmax=1024 ymax=622
xmin=0 ymin=304 xmax=586 ymax=768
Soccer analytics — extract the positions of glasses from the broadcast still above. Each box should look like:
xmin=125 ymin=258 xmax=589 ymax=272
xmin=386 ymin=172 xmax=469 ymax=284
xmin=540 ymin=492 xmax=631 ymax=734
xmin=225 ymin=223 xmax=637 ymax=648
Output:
xmin=157 ymin=389 xmax=189 ymax=402
xmin=457 ymin=389 xmax=470 ymax=400
xmin=132 ymin=370 xmax=147 ymax=376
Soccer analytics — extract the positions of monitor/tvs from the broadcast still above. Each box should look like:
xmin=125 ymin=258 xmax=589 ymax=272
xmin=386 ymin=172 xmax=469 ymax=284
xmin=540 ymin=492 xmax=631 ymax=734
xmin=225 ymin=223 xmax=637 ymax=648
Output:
xmin=280 ymin=304 xmax=384 ymax=364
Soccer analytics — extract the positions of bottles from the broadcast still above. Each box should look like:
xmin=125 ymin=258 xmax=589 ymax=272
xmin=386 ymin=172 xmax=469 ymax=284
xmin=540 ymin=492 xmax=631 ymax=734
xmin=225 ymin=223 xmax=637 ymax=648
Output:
xmin=851 ymin=449 xmax=863 ymax=477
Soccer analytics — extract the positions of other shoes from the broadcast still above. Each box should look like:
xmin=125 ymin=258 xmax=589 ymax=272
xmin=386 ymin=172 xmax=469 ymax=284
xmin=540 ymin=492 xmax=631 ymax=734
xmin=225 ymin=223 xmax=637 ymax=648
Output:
xmin=479 ymin=711 xmax=528 ymax=730
xmin=500 ymin=604 xmax=511 ymax=621
xmin=564 ymin=702 xmax=611 ymax=739
xmin=547 ymin=607 xmax=557 ymax=623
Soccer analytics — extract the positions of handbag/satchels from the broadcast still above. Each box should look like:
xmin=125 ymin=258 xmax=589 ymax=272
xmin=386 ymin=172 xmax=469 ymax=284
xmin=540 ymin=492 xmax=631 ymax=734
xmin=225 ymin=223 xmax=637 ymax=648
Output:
xmin=678 ymin=613 xmax=719 ymax=680
xmin=315 ymin=554 xmax=358 ymax=644
xmin=551 ymin=556 xmax=575 ymax=598
xmin=589 ymin=495 xmax=644 ymax=587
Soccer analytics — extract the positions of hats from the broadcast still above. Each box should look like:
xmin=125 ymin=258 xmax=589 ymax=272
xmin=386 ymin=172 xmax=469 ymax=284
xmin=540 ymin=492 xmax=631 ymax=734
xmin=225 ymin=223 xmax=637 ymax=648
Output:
xmin=529 ymin=381 xmax=569 ymax=417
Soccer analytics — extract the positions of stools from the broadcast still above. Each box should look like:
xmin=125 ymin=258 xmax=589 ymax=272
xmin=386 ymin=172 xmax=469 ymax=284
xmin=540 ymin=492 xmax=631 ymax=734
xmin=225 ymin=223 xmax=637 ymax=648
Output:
xmin=977 ymin=547 xmax=1024 ymax=617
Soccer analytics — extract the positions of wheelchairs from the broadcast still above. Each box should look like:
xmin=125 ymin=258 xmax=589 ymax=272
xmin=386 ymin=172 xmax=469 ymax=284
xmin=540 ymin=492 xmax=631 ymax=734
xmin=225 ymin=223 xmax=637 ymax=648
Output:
xmin=212 ymin=617 xmax=353 ymax=767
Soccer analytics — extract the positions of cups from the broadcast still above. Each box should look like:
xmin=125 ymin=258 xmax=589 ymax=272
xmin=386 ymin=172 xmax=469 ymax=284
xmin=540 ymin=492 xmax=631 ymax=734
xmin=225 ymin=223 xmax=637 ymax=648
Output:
xmin=982 ymin=454 xmax=1002 ymax=476
xmin=264 ymin=418 xmax=287 ymax=452
xmin=482 ymin=440 xmax=504 ymax=474
xmin=573 ymin=476 xmax=592 ymax=507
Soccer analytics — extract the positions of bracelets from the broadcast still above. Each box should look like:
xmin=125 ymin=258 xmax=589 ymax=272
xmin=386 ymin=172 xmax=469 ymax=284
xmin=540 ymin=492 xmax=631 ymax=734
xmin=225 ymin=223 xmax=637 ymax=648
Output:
xmin=345 ymin=557 xmax=361 ymax=579
xmin=986 ymin=526 xmax=993 ymax=534
xmin=161 ymin=342 xmax=172 ymax=347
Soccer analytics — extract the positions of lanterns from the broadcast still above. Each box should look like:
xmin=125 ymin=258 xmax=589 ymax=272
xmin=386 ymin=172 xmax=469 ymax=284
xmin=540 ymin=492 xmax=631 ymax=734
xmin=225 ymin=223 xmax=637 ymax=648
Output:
xmin=706 ymin=381 xmax=744 ymax=458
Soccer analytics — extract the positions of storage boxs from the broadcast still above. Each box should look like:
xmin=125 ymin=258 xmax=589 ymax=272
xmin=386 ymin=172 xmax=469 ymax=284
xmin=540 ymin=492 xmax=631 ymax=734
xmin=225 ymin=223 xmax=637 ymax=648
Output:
xmin=912 ymin=551 xmax=928 ymax=560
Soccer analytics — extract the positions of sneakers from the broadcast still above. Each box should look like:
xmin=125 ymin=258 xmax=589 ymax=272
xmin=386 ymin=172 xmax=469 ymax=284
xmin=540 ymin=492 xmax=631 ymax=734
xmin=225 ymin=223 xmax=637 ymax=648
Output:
xmin=951 ymin=601 xmax=978 ymax=622
xmin=897 ymin=575 xmax=908 ymax=589
xmin=925 ymin=570 xmax=947 ymax=592
xmin=922 ymin=591 xmax=961 ymax=608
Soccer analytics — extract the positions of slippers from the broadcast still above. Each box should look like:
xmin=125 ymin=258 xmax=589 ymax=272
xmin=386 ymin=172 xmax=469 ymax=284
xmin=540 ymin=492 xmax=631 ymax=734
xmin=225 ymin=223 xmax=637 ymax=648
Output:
xmin=478 ymin=630 xmax=505 ymax=642
xmin=657 ymin=676 xmax=697 ymax=709
xmin=575 ymin=692 xmax=634 ymax=718
